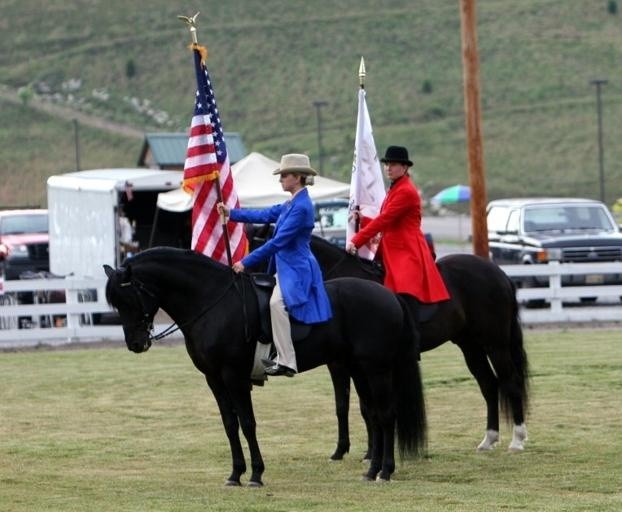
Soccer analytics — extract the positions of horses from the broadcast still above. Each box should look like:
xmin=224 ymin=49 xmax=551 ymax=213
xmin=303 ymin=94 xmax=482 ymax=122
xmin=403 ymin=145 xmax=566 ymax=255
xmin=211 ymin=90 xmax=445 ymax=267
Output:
xmin=245 ymin=223 xmax=533 ymax=463
xmin=103 ymin=246 xmax=427 ymax=487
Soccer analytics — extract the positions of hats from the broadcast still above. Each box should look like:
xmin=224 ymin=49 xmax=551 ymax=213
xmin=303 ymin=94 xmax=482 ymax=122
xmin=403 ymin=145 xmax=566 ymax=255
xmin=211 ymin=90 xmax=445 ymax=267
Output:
xmin=380 ymin=146 xmax=414 ymax=167
xmin=273 ymin=153 xmax=317 ymax=175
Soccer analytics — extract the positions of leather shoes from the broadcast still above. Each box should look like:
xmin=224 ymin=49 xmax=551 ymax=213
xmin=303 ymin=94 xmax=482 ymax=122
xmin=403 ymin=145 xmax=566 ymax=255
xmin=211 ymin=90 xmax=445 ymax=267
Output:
xmin=264 ymin=365 xmax=294 ymax=377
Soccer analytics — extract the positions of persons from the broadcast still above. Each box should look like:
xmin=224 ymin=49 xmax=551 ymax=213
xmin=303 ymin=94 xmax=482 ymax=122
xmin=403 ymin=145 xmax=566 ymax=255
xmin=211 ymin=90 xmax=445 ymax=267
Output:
xmin=217 ymin=153 xmax=333 ymax=378
xmin=347 ymin=146 xmax=452 ymax=304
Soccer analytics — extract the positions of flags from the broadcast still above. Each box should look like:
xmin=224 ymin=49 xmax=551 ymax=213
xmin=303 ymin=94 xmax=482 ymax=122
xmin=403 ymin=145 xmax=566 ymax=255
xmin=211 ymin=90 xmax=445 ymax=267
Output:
xmin=181 ymin=43 xmax=250 ymax=269
xmin=347 ymin=90 xmax=386 ymax=261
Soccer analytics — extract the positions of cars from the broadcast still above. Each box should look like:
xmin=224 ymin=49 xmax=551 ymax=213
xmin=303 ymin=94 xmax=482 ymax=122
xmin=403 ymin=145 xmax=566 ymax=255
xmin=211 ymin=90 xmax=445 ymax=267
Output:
xmin=311 ymin=200 xmax=436 ymax=263
xmin=485 ymin=198 xmax=621 ymax=309
xmin=1 ymin=208 xmax=50 ymax=281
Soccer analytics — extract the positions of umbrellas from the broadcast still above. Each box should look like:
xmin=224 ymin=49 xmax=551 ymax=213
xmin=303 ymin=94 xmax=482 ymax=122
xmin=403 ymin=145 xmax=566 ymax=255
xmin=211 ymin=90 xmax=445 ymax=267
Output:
xmin=432 ymin=184 xmax=471 ymax=238
